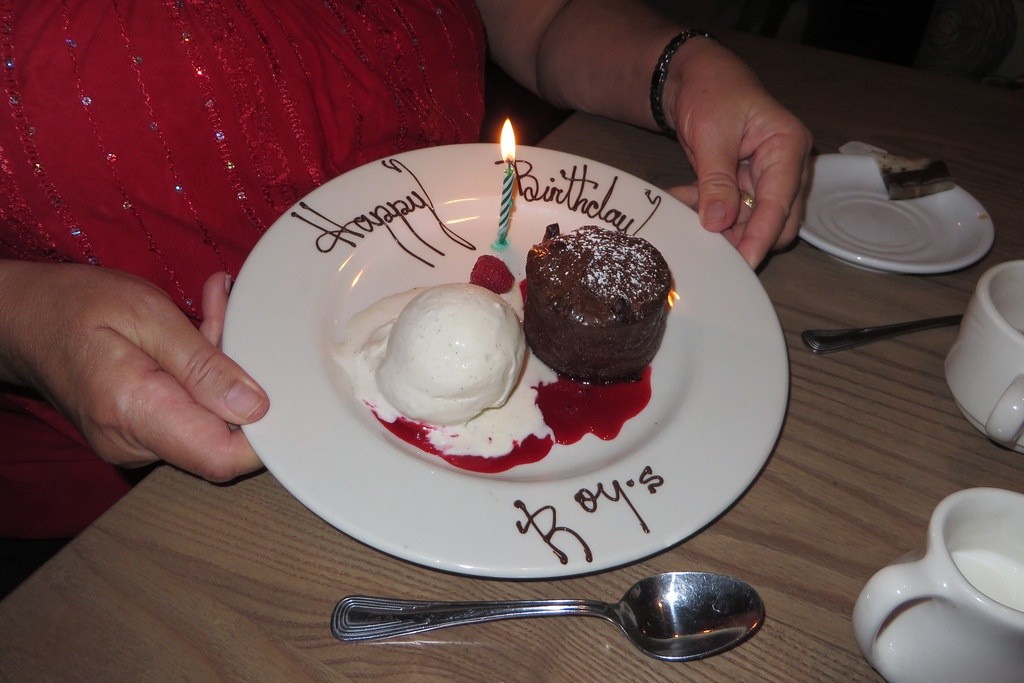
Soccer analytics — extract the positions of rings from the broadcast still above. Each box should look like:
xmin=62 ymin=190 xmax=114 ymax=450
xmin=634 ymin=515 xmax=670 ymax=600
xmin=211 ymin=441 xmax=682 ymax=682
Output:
xmin=738 ymin=188 xmax=757 ymax=212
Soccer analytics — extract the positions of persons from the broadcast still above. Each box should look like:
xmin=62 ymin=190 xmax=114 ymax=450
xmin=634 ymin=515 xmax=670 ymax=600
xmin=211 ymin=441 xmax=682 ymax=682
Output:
xmin=0 ymin=0 xmax=815 ymax=538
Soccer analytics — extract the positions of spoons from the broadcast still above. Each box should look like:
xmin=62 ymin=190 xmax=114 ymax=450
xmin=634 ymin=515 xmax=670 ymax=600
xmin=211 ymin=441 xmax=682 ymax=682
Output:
xmin=329 ymin=571 xmax=766 ymax=663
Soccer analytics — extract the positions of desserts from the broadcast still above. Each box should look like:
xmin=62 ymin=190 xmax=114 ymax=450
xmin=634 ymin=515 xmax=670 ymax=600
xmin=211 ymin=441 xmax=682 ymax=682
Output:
xmin=521 ymin=223 xmax=672 ymax=384
xmin=373 ymin=282 xmax=526 ymax=425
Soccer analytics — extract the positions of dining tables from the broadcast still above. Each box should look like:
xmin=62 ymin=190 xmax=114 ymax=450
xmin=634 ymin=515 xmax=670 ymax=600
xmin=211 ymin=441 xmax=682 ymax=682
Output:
xmin=1 ymin=29 xmax=1024 ymax=683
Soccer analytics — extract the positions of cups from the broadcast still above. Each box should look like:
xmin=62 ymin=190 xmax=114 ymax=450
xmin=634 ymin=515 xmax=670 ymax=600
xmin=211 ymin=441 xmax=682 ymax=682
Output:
xmin=943 ymin=258 xmax=1024 ymax=454
xmin=850 ymin=486 xmax=1024 ymax=683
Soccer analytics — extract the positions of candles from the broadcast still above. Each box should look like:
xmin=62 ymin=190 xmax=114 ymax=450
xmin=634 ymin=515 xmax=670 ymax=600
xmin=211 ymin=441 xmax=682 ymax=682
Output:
xmin=494 ymin=117 xmax=515 ymax=250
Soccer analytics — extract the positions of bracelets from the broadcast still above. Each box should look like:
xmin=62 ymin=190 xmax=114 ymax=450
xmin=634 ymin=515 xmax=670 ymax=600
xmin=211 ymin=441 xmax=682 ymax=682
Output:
xmin=650 ymin=28 xmax=718 ymax=144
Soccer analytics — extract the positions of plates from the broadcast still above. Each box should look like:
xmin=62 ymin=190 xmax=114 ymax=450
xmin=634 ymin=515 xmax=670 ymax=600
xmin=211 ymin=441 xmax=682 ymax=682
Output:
xmin=220 ymin=141 xmax=789 ymax=583
xmin=797 ymin=153 xmax=995 ymax=274
